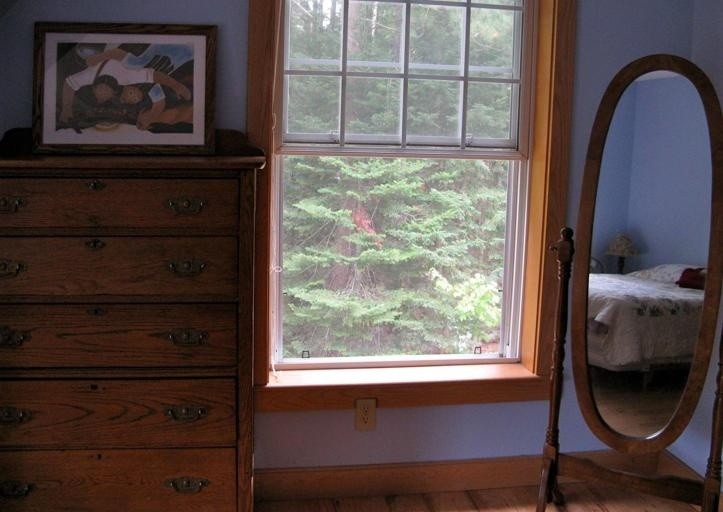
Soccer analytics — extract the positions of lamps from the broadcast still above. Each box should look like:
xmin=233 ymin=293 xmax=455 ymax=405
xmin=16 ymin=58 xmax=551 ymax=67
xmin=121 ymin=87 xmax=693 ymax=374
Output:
xmin=604 ymin=231 xmax=640 ymax=277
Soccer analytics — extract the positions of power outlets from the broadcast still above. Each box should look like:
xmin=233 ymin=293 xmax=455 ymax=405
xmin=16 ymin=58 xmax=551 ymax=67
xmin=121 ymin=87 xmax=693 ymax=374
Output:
xmin=354 ymin=397 xmax=377 ymax=431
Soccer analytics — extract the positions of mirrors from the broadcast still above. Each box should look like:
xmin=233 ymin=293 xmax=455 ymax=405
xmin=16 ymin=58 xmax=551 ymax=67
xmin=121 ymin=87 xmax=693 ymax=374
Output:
xmin=532 ymin=53 xmax=723 ymax=509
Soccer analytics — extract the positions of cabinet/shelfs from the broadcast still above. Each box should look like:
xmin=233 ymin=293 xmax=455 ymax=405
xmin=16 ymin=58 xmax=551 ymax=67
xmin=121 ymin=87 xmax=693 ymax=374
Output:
xmin=0 ymin=125 xmax=268 ymax=510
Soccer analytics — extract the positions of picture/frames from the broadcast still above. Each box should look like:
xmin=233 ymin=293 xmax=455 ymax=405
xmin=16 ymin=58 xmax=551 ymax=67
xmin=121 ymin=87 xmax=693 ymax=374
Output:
xmin=32 ymin=18 xmax=218 ymax=156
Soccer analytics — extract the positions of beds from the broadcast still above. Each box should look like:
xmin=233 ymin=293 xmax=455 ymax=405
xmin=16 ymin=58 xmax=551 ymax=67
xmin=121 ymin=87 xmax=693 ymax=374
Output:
xmin=589 ymin=269 xmax=708 ymax=391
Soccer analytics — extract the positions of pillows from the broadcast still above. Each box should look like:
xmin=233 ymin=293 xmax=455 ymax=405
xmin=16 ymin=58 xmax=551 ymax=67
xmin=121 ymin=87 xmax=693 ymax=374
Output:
xmin=625 ymin=263 xmax=707 ymax=289
